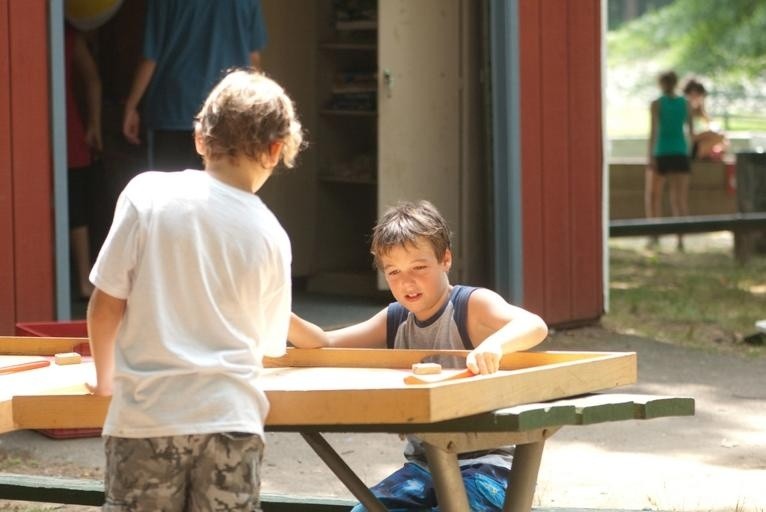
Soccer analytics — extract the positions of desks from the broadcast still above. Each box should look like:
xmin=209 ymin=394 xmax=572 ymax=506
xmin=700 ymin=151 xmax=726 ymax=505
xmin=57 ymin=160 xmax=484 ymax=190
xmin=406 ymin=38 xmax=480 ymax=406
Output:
xmin=0 ymin=393 xmax=695 ymax=512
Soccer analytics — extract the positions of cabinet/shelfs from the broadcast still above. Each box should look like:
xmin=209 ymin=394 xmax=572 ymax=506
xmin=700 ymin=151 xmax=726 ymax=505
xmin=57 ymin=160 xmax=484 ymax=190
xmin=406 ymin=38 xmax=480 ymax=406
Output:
xmin=265 ymin=0 xmax=483 ymax=302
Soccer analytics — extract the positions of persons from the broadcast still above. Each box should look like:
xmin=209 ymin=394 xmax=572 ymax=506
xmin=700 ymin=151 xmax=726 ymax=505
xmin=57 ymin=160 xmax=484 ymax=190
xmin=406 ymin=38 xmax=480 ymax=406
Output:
xmin=286 ymin=195 xmax=550 ymax=512
xmin=62 ymin=13 xmax=105 ymax=303
xmin=681 ymin=75 xmax=725 ymax=173
xmin=121 ymin=0 xmax=269 ymax=176
xmin=639 ymin=68 xmax=697 ymax=255
xmin=83 ymin=59 xmax=308 ymax=512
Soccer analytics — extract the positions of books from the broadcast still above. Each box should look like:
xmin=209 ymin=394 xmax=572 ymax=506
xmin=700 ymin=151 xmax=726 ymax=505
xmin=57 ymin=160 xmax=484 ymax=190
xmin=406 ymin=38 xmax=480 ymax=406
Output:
xmin=319 ymin=1 xmax=378 ymax=183
